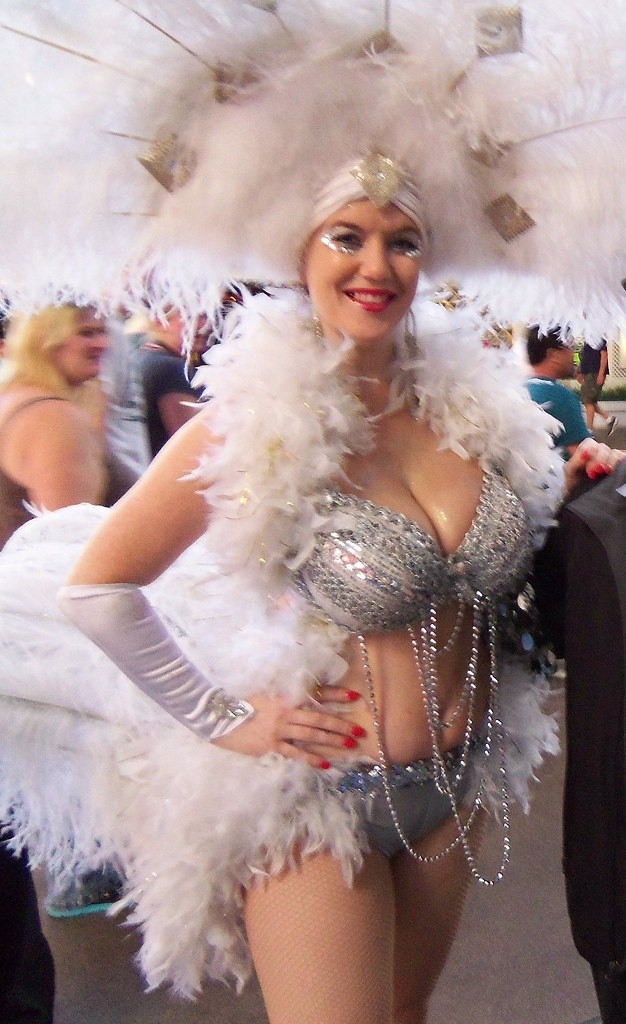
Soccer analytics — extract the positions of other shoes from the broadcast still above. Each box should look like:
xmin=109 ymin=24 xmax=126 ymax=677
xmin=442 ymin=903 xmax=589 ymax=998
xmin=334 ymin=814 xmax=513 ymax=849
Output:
xmin=46 ymin=863 xmax=134 ymax=916
xmin=606 ymin=416 xmax=619 ymax=436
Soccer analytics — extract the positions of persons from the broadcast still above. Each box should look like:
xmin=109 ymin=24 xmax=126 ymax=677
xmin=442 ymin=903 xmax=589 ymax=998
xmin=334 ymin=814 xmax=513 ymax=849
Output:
xmin=0 ymin=151 xmax=626 ymax=1024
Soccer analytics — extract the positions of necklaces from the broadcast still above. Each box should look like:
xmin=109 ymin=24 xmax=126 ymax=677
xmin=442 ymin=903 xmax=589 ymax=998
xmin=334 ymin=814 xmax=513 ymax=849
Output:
xmin=311 ymin=317 xmax=388 ymax=387
xmin=150 ymin=339 xmax=175 ymax=355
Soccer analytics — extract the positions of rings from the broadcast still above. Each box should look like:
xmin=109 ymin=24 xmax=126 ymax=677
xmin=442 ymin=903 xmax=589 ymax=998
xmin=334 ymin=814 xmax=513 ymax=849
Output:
xmin=315 ymin=685 xmax=323 ymax=702
